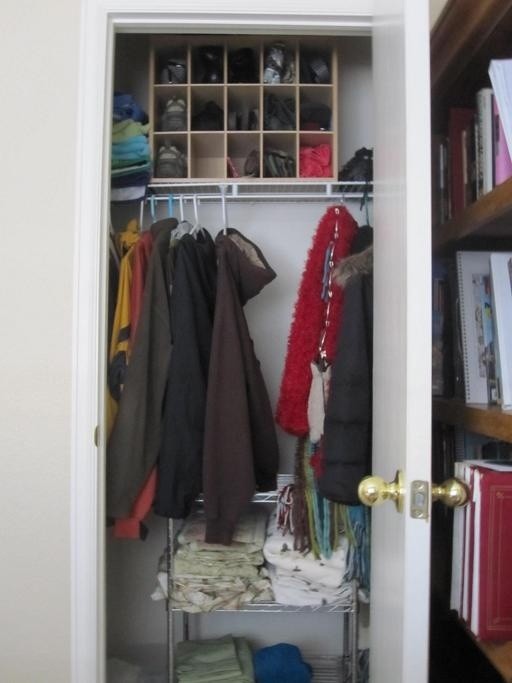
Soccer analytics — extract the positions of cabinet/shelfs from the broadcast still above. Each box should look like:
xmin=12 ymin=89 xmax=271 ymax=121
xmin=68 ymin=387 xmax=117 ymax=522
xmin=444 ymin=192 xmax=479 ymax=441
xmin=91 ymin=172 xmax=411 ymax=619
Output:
xmin=147 ymin=33 xmax=340 ymax=185
xmin=162 ymin=473 xmax=358 ymax=683
xmin=431 ymin=0 xmax=512 ymax=683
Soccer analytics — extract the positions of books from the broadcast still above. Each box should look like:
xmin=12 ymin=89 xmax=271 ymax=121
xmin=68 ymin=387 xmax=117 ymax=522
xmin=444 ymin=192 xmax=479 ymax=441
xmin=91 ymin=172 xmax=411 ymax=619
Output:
xmin=434 ymin=251 xmax=512 ymax=415
xmin=435 ymin=56 xmax=512 ymax=227
xmin=450 ymin=458 xmax=512 ymax=643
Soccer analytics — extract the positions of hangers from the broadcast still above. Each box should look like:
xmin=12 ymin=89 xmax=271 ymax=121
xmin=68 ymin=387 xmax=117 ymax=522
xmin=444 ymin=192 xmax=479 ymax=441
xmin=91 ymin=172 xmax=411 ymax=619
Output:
xmin=131 ymin=190 xmax=374 ymax=244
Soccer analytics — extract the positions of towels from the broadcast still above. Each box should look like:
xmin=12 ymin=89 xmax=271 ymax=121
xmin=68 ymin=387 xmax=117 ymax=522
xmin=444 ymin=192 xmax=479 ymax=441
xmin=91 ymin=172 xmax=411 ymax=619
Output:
xmin=150 ymin=520 xmax=356 ymax=683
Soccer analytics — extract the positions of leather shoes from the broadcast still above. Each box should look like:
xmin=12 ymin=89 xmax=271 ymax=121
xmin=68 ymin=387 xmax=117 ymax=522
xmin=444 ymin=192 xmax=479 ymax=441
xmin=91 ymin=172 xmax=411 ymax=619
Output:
xmin=199 ymin=45 xmax=222 ymax=83
xmin=228 ymin=47 xmax=259 ymax=82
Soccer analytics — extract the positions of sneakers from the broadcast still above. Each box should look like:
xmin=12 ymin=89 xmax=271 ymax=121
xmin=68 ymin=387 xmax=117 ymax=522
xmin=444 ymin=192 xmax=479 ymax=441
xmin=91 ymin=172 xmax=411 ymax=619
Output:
xmin=263 ymin=44 xmax=295 ymax=83
xmin=158 ymin=53 xmax=186 ymax=83
xmin=158 ymin=139 xmax=185 ymax=177
xmin=162 ymin=95 xmax=187 ymax=131
